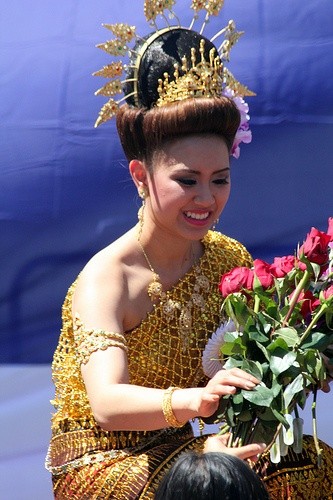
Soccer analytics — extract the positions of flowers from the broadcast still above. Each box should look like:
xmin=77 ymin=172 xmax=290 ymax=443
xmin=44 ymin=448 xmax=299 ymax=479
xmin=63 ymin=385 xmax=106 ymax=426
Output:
xmin=214 ymin=216 xmax=333 ymax=471
xmin=222 ymin=84 xmax=252 ymax=160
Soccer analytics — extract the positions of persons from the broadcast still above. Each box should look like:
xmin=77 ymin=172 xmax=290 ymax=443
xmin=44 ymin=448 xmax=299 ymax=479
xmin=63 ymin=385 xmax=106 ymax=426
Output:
xmin=154 ymin=449 xmax=273 ymax=499
xmin=44 ymin=28 xmax=333 ymax=499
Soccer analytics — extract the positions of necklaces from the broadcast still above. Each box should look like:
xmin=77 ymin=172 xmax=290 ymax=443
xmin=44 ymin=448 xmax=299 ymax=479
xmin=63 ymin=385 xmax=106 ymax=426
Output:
xmin=135 ymin=205 xmax=198 ymax=307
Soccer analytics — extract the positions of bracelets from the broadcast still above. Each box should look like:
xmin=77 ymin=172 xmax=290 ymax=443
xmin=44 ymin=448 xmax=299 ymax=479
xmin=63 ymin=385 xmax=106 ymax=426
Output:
xmin=162 ymin=386 xmax=189 ymax=430
